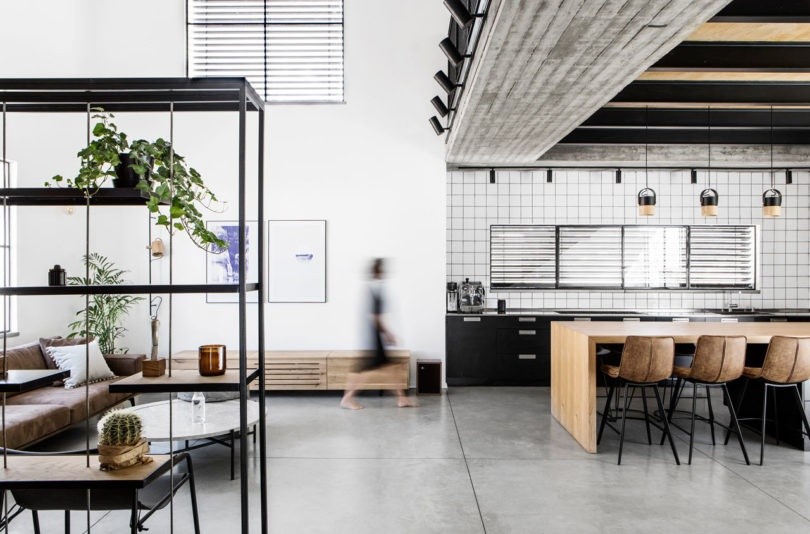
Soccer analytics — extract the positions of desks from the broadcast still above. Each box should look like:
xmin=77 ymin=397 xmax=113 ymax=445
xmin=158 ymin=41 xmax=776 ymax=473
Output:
xmin=98 ymin=398 xmax=260 ymax=482
xmin=549 ymin=320 xmax=809 ymax=452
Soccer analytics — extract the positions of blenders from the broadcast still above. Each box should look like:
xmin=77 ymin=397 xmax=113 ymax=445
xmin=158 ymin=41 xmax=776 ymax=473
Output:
xmin=446 ymin=282 xmax=458 ymax=312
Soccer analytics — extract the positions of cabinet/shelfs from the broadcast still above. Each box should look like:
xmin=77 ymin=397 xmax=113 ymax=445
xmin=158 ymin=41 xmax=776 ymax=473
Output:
xmin=0 ymin=74 xmax=268 ymax=533
xmin=442 ymin=308 xmax=807 ymax=385
xmin=165 ymin=348 xmax=412 ymax=394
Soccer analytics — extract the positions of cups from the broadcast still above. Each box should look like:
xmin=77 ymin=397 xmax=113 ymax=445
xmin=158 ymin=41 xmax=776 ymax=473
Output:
xmin=198 ymin=344 xmax=226 ymax=376
xmin=498 ymin=300 xmax=505 ymax=313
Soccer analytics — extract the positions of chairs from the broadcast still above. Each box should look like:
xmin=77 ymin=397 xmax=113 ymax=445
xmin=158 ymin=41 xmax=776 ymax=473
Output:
xmin=596 ymin=334 xmax=682 ymax=469
xmin=721 ymin=336 xmax=810 ymax=465
xmin=659 ymin=334 xmax=754 ymax=467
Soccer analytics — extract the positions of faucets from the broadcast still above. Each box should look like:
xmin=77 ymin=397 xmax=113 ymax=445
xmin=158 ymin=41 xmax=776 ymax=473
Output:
xmin=727 ymin=288 xmax=741 ymax=312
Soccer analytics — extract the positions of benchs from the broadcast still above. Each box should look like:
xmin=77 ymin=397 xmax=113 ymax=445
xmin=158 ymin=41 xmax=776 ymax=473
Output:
xmin=0 ymin=336 xmax=147 ymax=450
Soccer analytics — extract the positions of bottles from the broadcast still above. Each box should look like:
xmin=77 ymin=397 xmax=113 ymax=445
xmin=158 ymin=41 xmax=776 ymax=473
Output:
xmin=48 ymin=265 xmax=65 ymax=286
xmin=191 ymin=392 xmax=206 ymax=424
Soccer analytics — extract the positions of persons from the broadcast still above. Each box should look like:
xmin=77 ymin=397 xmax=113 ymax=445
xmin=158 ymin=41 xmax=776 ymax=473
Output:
xmin=339 ymin=255 xmax=422 ymax=410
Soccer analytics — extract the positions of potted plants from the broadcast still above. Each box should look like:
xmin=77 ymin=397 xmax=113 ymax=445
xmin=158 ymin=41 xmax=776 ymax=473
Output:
xmin=95 ymin=408 xmax=149 ymax=467
xmin=42 ymin=104 xmax=229 ymax=255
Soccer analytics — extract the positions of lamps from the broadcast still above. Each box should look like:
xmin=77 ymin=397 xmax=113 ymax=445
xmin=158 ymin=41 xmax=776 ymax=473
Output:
xmin=638 ymin=104 xmax=656 ymax=217
xmin=762 ymin=105 xmax=782 ymax=217
xmin=700 ymin=104 xmax=720 ymax=216
xmin=428 ymin=0 xmax=485 ymax=135
xmin=489 ymin=167 xmax=793 ymax=184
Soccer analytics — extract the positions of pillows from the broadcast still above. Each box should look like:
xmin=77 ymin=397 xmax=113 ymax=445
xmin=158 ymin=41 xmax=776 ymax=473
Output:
xmin=40 ymin=332 xmax=120 ymax=390
